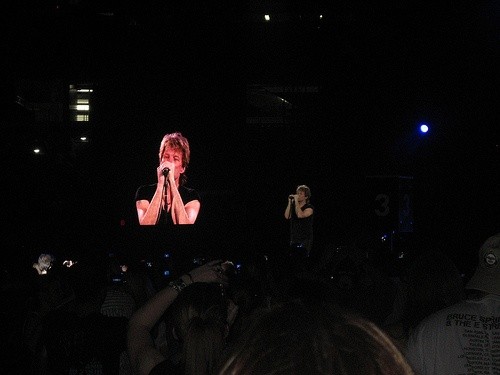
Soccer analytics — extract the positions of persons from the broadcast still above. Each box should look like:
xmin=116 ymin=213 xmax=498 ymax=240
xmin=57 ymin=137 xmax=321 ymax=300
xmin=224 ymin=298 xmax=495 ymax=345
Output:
xmin=1 ymin=234 xmax=500 ymax=375
xmin=134 ymin=132 xmax=201 ymax=226
xmin=284 ymin=185 xmax=315 ymax=258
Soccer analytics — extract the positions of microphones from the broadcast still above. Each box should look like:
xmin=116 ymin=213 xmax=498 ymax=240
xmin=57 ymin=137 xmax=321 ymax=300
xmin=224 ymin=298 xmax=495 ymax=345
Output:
xmin=162 ymin=168 xmax=169 ymax=174
xmin=289 ymin=195 xmax=295 ymax=199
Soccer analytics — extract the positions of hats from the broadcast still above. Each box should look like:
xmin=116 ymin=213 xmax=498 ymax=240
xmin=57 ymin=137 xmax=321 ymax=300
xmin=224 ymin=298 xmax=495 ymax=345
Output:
xmin=472 ymin=236 xmax=500 ymax=295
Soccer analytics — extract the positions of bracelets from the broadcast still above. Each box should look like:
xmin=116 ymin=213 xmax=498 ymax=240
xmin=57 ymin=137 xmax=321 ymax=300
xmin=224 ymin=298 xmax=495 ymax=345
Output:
xmin=174 ymin=278 xmax=186 ymax=291
xmin=184 ymin=272 xmax=195 ymax=285
xmin=167 ymin=283 xmax=180 ymax=295
